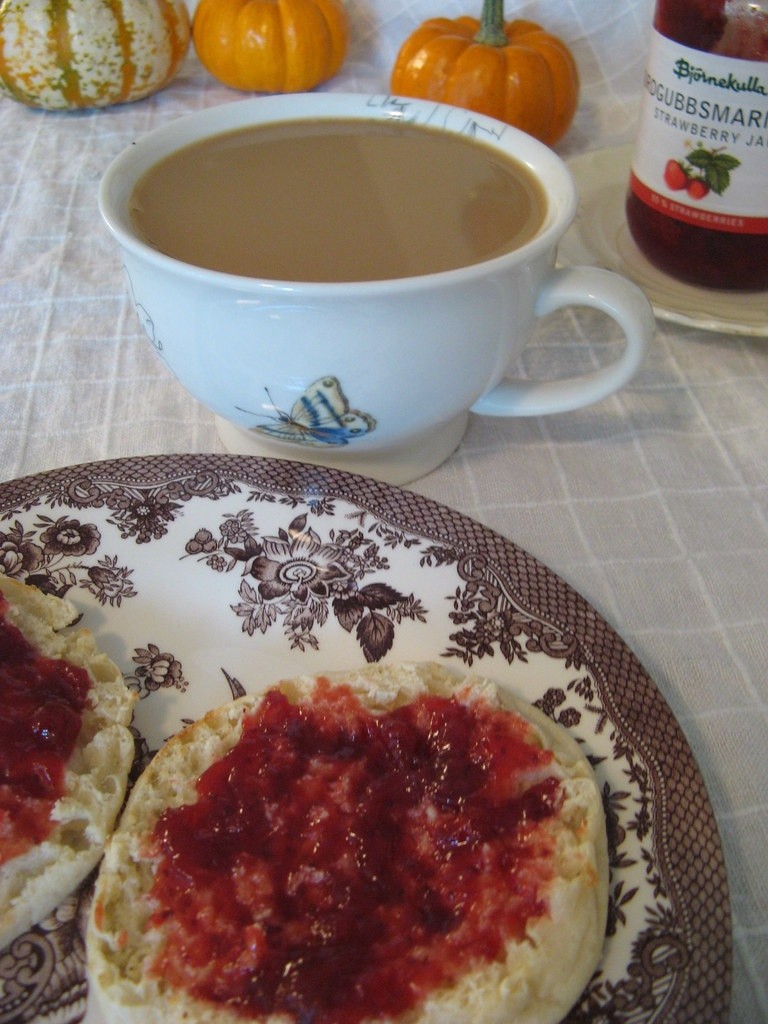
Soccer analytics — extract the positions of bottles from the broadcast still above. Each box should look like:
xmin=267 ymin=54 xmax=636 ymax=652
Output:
xmin=626 ymin=0 xmax=768 ymax=295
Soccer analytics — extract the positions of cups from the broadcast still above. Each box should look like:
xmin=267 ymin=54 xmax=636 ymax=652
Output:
xmin=97 ymin=93 xmax=655 ymax=487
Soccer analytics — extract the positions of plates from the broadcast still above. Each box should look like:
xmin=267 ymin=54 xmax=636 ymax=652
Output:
xmin=0 ymin=452 xmax=728 ymax=1024
xmin=555 ymin=142 xmax=768 ymax=340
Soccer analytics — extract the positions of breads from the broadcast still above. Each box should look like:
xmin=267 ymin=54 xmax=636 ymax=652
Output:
xmin=90 ymin=660 xmax=603 ymax=1024
xmin=0 ymin=575 xmax=133 ymax=954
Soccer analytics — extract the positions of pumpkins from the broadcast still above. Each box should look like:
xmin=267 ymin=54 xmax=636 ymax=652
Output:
xmin=0 ymin=0 xmax=191 ymax=112
xmin=190 ymin=0 xmax=351 ymax=94
xmin=391 ymin=0 xmax=578 ymax=154
